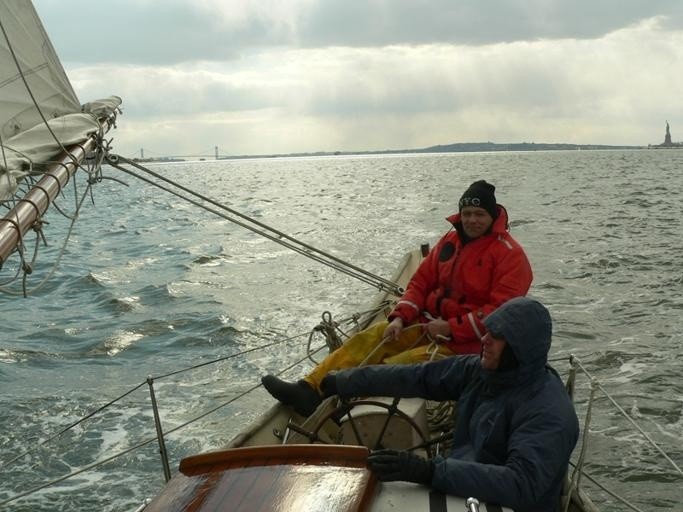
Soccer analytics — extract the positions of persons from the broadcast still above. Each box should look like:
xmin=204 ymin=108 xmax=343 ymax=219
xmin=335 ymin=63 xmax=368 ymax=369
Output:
xmin=261 ymin=178 xmax=535 ymax=418
xmin=316 ymin=296 xmax=579 ymax=511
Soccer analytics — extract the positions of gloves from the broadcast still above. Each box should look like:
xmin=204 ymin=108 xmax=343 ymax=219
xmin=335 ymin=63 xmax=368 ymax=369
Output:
xmin=319 ymin=374 xmax=338 ymax=395
xmin=365 ymin=448 xmax=436 ymax=486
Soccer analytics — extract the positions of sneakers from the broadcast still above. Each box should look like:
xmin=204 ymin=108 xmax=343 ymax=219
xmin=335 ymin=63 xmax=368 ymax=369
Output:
xmin=260 ymin=373 xmax=322 ymax=419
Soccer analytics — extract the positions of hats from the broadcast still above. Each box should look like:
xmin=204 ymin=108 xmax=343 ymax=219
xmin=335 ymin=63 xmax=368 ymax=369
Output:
xmin=458 ymin=179 xmax=498 ymax=221
